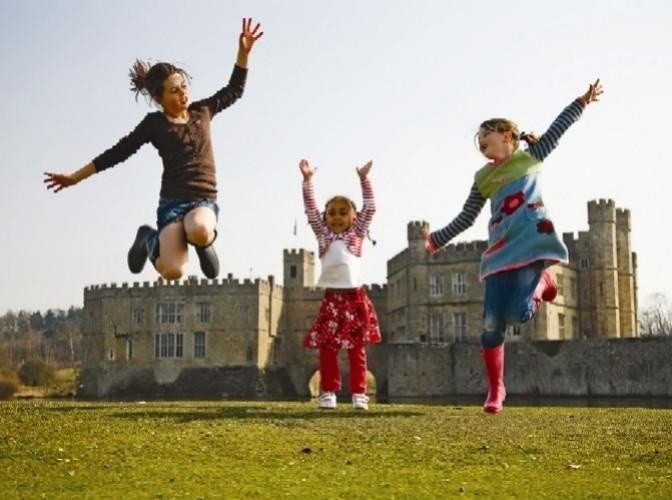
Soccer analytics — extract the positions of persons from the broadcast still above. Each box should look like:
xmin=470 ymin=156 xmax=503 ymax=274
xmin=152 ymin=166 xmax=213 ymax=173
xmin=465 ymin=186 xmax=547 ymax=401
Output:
xmin=417 ymin=78 xmax=607 ymax=416
xmin=298 ymin=157 xmax=384 ymax=412
xmin=44 ymin=12 xmax=265 ymax=281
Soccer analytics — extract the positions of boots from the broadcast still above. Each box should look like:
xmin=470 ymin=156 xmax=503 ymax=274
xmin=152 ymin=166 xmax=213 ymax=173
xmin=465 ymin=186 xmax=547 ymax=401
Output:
xmin=187 ymin=228 xmax=219 ymax=279
xmin=483 ymin=342 xmax=506 ymax=412
xmin=532 ymin=268 xmax=558 ymax=310
xmin=128 ymin=226 xmax=160 ymax=273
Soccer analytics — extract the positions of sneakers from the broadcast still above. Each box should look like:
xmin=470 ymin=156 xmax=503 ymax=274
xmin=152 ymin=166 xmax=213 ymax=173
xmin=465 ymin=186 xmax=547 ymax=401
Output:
xmin=352 ymin=392 xmax=370 ymax=410
xmin=319 ymin=392 xmax=337 ymax=409
